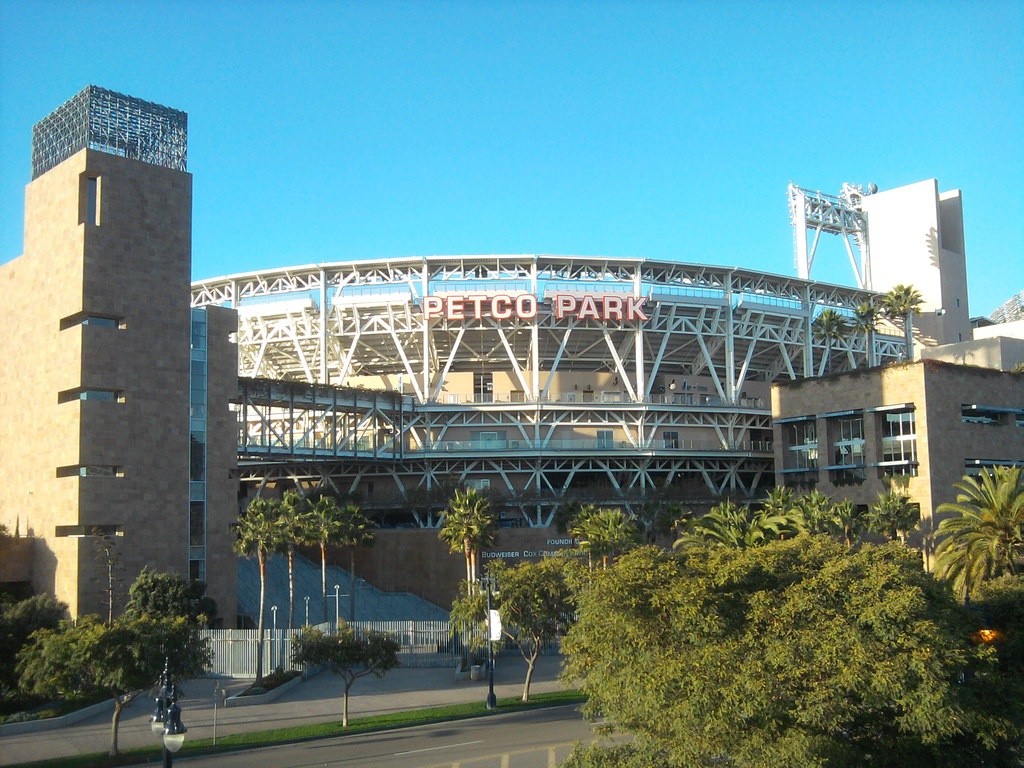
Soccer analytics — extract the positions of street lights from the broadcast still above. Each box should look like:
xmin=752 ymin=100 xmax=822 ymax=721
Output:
xmin=148 ymin=657 xmax=187 ymax=768
xmin=479 ymin=568 xmax=500 ymax=708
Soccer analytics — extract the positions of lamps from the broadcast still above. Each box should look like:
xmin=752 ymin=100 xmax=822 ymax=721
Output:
xmin=669 ymin=379 xmax=676 ymax=390
